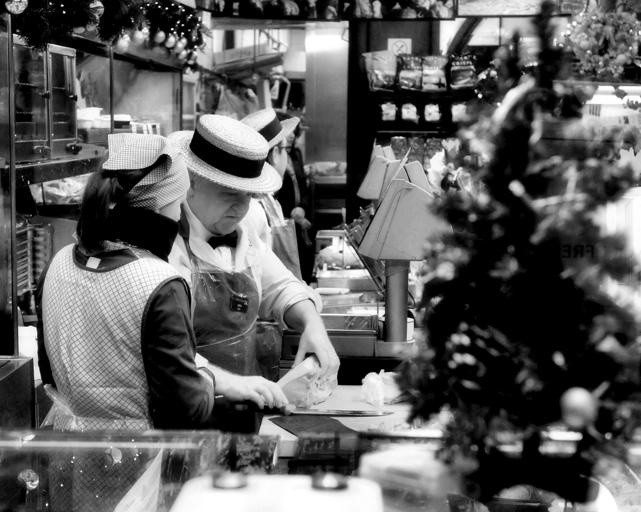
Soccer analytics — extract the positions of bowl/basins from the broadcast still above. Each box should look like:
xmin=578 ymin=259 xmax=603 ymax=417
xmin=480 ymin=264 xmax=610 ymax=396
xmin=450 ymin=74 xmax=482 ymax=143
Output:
xmin=75 ymin=107 xmax=131 ymax=130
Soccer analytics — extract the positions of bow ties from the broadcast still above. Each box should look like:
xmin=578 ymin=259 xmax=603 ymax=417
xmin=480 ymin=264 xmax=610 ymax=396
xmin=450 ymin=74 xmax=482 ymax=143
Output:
xmin=206 ymin=230 xmax=239 ymax=251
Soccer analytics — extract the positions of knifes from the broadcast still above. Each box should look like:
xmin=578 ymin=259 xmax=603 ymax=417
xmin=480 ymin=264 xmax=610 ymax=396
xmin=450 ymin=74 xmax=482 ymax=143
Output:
xmin=282 ymin=409 xmax=392 ymax=418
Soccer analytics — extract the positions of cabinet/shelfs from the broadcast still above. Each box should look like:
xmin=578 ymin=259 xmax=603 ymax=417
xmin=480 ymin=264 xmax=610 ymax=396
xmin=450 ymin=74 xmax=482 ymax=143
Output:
xmin=10 ymin=34 xmax=81 ymax=162
xmin=362 ymin=78 xmax=481 ymax=140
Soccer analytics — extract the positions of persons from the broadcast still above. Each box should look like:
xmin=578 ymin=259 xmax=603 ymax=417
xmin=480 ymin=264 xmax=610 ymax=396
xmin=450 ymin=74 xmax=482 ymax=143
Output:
xmin=273 ymin=113 xmax=318 ymax=285
xmin=238 ymin=107 xmax=303 ymax=283
xmin=35 ymin=132 xmax=217 ymax=511
xmin=158 ymin=109 xmax=341 ymax=410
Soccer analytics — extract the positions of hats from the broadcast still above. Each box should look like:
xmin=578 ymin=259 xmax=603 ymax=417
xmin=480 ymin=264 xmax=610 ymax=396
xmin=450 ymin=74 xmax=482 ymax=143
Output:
xmin=238 ymin=107 xmax=301 ymax=150
xmin=166 ymin=114 xmax=284 ymax=195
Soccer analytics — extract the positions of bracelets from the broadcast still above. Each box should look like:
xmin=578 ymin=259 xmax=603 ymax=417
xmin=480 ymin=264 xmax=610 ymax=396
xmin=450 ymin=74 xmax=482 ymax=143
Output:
xmin=196 ymin=366 xmax=216 ymax=389
